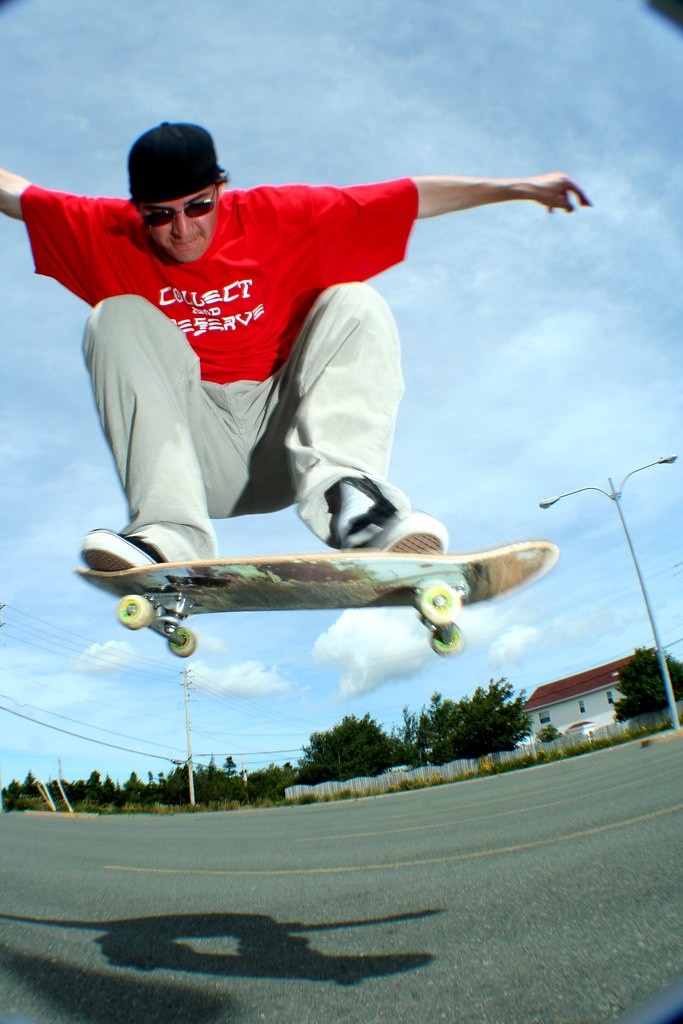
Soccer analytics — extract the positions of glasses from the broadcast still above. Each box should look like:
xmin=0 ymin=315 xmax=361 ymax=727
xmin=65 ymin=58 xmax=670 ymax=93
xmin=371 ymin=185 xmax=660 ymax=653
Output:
xmin=142 ymin=183 xmax=216 ymax=227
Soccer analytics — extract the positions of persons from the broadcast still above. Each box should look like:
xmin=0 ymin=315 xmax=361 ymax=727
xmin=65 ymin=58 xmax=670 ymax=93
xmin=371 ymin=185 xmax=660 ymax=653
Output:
xmin=0 ymin=122 xmax=592 ymax=571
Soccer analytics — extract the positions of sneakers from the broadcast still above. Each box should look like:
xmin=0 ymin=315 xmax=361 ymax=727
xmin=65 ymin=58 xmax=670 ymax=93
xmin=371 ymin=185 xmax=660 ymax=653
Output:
xmin=80 ymin=529 xmax=165 ymax=571
xmin=344 ymin=503 xmax=449 ymax=554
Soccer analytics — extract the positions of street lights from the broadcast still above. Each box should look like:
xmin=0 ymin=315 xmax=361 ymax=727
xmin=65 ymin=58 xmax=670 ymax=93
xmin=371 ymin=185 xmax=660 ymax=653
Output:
xmin=540 ymin=452 xmax=682 ymax=732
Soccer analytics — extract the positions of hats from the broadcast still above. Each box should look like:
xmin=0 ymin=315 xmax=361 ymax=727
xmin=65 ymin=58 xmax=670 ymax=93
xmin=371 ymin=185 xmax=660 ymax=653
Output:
xmin=128 ymin=121 xmax=228 ymax=204
